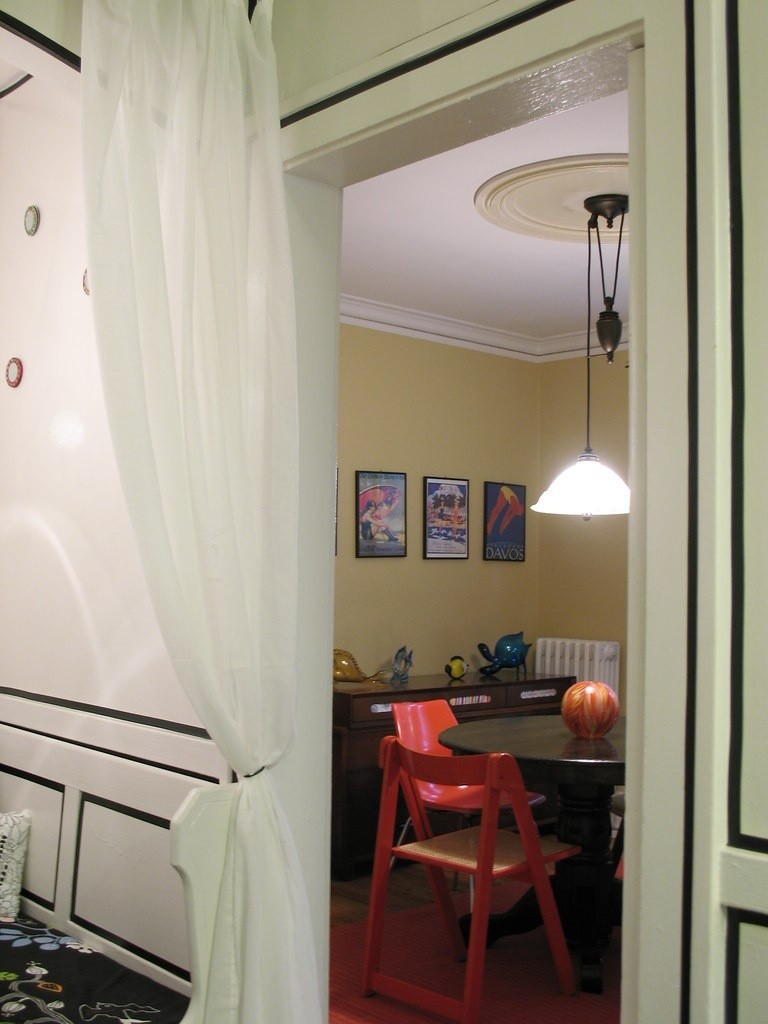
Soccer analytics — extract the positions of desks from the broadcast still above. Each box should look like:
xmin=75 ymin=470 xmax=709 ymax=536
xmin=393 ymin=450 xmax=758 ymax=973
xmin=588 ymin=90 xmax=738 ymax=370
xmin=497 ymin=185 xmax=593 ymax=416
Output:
xmin=431 ymin=716 xmax=628 ymax=889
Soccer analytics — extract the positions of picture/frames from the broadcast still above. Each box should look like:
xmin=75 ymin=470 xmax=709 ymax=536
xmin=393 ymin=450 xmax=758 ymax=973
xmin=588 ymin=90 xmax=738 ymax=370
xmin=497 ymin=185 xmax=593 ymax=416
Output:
xmin=354 ymin=469 xmax=527 ymax=562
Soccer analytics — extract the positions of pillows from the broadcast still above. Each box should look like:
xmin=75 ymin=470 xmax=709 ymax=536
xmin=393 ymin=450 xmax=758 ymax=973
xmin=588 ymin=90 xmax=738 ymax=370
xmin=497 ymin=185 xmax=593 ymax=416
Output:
xmin=0 ymin=807 xmax=30 ymax=923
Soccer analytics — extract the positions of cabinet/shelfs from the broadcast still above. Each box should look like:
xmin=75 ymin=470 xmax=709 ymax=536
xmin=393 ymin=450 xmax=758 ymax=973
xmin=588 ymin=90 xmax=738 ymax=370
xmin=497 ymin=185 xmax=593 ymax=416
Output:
xmin=329 ymin=668 xmax=579 ymax=881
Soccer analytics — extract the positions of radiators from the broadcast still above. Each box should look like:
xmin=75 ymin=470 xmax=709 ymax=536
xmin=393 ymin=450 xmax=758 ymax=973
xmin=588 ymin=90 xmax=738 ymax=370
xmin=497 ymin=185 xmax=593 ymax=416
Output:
xmin=535 ymin=637 xmax=620 ymax=698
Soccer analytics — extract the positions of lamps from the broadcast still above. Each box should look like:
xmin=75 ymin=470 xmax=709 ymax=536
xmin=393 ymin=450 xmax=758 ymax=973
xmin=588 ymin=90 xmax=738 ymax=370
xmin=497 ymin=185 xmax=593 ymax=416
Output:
xmin=528 ymin=195 xmax=637 ymax=520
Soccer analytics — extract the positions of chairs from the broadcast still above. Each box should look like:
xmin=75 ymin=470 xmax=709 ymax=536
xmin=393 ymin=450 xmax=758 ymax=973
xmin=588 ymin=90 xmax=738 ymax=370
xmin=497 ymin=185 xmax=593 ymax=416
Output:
xmin=386 ymin=699 xmax=550 ymax=916
xmin=360 ymin=730 xmax=580 ymax=1024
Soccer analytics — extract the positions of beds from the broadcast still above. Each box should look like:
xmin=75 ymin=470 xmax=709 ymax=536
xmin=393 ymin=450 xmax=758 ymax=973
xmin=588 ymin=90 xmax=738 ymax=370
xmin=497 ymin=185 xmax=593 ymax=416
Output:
xmin=0 ymin=685 xmax=238 ymax=1024
